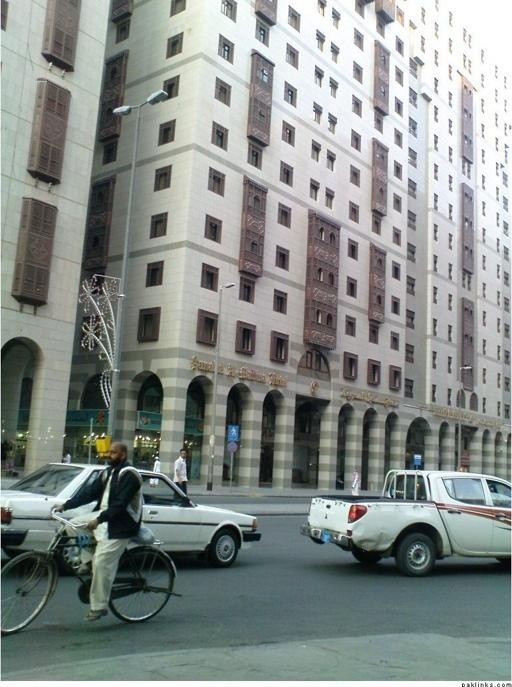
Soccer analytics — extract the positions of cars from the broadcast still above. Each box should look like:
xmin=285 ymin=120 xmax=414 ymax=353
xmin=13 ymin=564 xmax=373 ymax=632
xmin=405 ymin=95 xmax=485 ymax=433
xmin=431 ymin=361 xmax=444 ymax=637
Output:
xmin=1 ymin=462 xmax=261 ymax=575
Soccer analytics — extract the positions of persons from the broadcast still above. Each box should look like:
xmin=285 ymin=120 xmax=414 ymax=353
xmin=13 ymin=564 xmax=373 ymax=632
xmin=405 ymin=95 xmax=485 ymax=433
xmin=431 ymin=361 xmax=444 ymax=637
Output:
xmin=148 ymin=456 xmax=160 ymax=489
xmin=59 ymin=450 xmax=71 ymax=464
xmin=349 ymin=467 xmax=360 ymax=498
xmin=172 ymin=448 xmax=188 ymax=499
xmin=55 ymin=441 xmax=144 ymax=624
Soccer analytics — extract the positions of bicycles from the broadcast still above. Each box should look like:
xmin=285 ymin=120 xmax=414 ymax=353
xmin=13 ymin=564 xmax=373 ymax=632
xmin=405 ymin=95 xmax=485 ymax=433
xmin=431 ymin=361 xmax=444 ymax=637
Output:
xmin=1 ymin=504 xmax=183 ymax=636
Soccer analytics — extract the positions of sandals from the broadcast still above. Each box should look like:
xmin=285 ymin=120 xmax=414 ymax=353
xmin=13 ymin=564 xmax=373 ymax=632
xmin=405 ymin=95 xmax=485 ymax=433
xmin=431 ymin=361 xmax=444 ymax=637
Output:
xmin=84 ymin=609 xmax=107 ymax=621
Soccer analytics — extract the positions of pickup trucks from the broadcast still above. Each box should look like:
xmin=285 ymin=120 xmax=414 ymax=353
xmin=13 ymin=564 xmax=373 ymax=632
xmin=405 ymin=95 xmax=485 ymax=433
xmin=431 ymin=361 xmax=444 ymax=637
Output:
xmin=300 ymin=468 xmax=512 ymax=575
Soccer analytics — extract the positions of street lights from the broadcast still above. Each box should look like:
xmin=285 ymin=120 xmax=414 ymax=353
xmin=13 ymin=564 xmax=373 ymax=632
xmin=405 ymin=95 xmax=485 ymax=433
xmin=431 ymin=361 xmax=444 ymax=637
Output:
xmin=105 ymin=88 xmax=168 ymax=460
xmin=207 ymin=281 xmax=235 ymax=491
xmin=457 ymin=365 xmax=473 ymax=471
